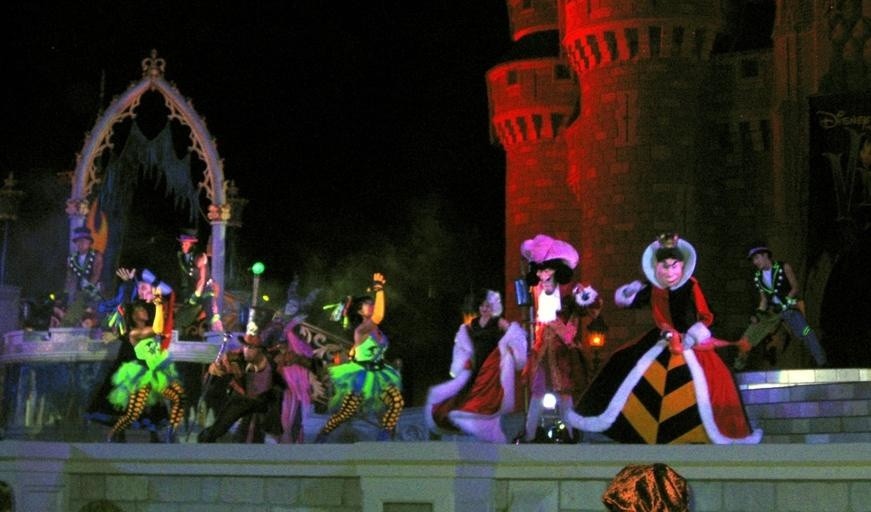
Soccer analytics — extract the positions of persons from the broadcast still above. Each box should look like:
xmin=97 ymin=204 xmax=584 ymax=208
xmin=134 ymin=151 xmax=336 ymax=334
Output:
xmin=174 ymin=233 xmax=225 ymax=340
xmin=105 ymin=267 xmax=189 ymax=442
xmin=515 ymin=231 xmax=604 ymax=442
xmin=196 ymin=333 xmax=276 ymax=443
xmin=567 ymin=233 xmax=762 ymax=445
xmin=730 ymin=245 xmax=831 ymax=372
xmin=425 ymin=287 xmax=528 ymax=443
xmin=313 ymin=271 xmax=406 ymax=443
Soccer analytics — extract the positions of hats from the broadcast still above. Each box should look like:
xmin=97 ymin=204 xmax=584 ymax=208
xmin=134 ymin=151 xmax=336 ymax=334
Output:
xmin=71 ymin=226 xmax=94 ymax=246
xmin=747 ymin=246 xmax=774 ymax=259
xmin=176 ymin=227 xmax=201 ymax=243
xmin=237 ymin=332 xmax=267 ymax=348
xmin=517 ymin=236 xmax=581 ymax=270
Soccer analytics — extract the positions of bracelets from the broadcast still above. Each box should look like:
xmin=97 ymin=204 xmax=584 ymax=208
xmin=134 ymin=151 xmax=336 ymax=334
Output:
xmin=152 ymin=300 xmax=164 ymax=305
xmin=372 ymin=280 xmax=384 ymax=286
xmin=373 ymin=286 xmax=385 ymax=292
